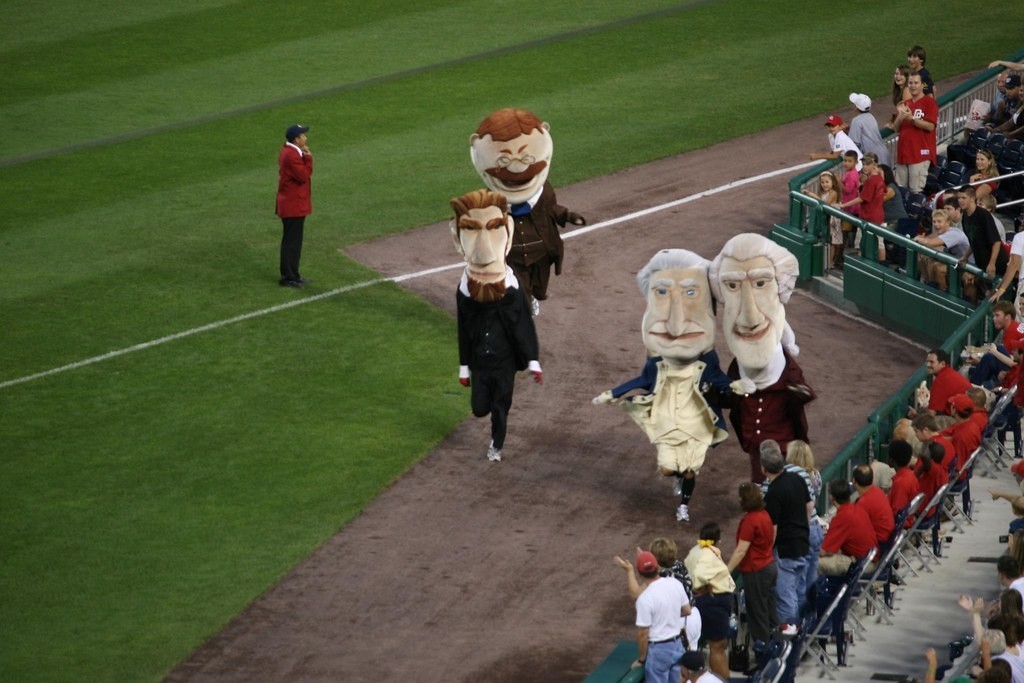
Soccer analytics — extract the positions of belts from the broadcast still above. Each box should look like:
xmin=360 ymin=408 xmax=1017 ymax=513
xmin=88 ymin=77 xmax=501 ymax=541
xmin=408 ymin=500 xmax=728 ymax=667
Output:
xmin=649 ymin=633 xmax=681 ymax=644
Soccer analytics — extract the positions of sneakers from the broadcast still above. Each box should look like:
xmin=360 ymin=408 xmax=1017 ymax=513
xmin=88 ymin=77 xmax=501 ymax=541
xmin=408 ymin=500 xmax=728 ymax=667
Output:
xmin=487 ymin=440 xmax=502 ymax=462
xmin=676 ymin=504 xmax=690 ymax=522
xmin=531 ymin=294 xmax=541 ymax=317
xmin=673 ymin=477 xmax=683 ymax=495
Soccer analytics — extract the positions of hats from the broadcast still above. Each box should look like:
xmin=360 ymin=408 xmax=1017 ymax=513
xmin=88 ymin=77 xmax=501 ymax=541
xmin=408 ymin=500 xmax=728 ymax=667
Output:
xmin=824 ymin=115 xmax=842 ymax=126
xmin=999 ymin=75 xmax=1021 ymax=87
xmin=860 ymin=151 xmax=878 ymax=163
xmin=1009 ymin=338 xmax=1024 ymax=351
xmin=636 ymin=551 xmax=657 ymax=572
xmin=286 ymin=124 xmax=309 ymax=141
xmin=948 ymin=394 xmax=974 ymax=415
xmin=849 ymin=93 xmax=871 ymax=112
xmin=677 ymin=652 xmax=704 ymax=669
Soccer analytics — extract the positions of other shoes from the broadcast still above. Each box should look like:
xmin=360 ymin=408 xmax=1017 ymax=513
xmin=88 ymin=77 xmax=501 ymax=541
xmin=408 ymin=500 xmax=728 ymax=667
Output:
xmin=282 ymin=277 xmax=310 ymax=289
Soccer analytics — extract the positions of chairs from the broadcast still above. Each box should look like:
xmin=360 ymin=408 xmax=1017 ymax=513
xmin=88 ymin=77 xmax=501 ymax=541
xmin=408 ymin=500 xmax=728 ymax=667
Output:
xmin=755 ymin=385 xmax=1018 ymax=683
xmin=886 ymin=127 xmax=1024 ymax=270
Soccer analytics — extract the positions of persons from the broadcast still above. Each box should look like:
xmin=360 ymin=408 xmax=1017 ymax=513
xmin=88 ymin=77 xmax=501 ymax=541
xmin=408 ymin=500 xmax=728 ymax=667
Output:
xmin=275 ymin=125 xmax=314 ymax=287
xmin=615 ymin=46 xmax=1024 ymax=683
xmin=449 ymin=109 xmax=816 ymax=521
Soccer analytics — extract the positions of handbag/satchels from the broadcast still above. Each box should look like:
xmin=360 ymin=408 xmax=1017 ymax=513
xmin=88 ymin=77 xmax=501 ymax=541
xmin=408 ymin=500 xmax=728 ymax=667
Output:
xmin=964 ymin=98 xmax=991 ymax=131
